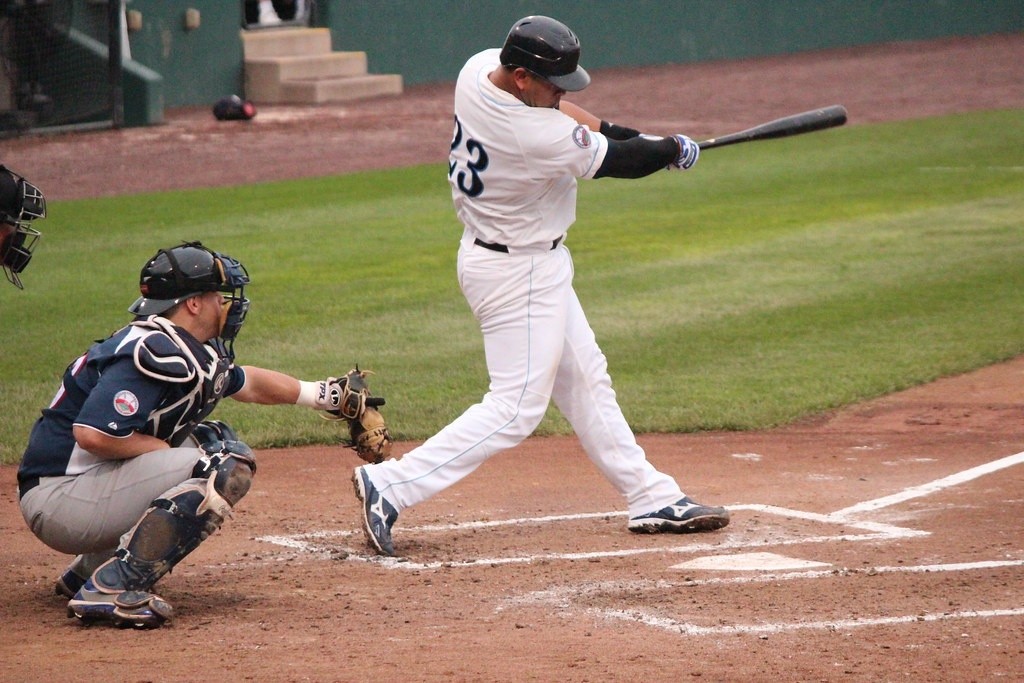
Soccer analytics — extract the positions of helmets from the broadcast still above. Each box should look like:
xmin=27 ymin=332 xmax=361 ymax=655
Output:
xmin=499 ymin=15 xmax=591 ymax=92
xmin=128 ymin=239 xmax=221 ymax=316
xmin=0 ymin=163 xmax=19 ymax=218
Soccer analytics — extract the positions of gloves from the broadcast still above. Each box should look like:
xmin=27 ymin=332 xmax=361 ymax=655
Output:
xmin=673 ymin=132 xmax=700 ymax=170
xmin=638 ymin=132 xmax=671 ymax=170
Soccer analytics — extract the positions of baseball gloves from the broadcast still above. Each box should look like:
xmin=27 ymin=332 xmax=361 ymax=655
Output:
xmin=320 ymin=363 xmax=393 ymax=465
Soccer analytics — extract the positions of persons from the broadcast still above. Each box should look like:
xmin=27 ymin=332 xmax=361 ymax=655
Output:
xmin=0 ymin=164 xmax=46 ymax=290
xmin=351 ymin=16 xmax=731 ymax=554
xmin=16 ymin=242 xmax=390 ymax=628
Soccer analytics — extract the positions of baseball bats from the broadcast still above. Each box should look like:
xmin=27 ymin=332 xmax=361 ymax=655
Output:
xmin=693 ymin=105 xmax=849 ymax=150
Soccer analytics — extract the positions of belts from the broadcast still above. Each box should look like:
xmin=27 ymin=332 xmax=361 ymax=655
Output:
xmin=474 ymin=235 xmax=563 ymax=253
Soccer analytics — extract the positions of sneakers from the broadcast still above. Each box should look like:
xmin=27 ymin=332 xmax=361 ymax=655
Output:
xmin=66 ymin=576 xmax=162 ymax=629
xmin=351 ymin=465 xmax=398 ymax=557
xmin=627 ymin=496 xmax=730 ymax=534
xmin=55 ymin=570 xmax=87 ymax=599
